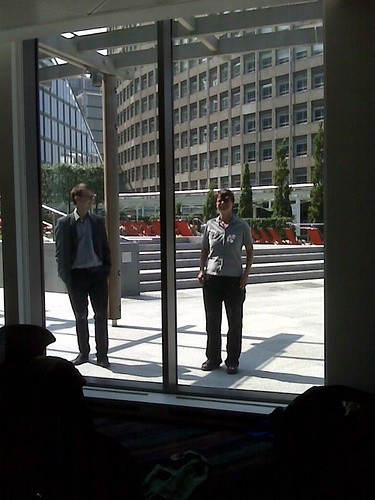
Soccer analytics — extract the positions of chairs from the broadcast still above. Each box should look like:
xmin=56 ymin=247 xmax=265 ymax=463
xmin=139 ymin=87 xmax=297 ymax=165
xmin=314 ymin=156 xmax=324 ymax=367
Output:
xmin=307 ymin=228 xmax=325 ymax=245
xmin=119 ymin=221 xmax=305 ymax=245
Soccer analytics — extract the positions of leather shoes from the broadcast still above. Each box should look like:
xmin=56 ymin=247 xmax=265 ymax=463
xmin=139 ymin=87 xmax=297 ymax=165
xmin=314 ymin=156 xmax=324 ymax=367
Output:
xmin=226 ymin=359 xmax=238 ymax=374
xmin=201 ymin=359 xmax=221 ymax=372
xmin=74 ymin=353 xmax=88 ymax=365
xmin=96 ymin=353 xmax=110 ymax=368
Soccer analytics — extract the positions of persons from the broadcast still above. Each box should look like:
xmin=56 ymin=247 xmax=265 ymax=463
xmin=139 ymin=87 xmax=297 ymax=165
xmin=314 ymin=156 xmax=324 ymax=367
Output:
xmin=198 ymin=189 xmax=254 ymax=374
xmin=54 ymin=186 xmax=112 ymax=368
